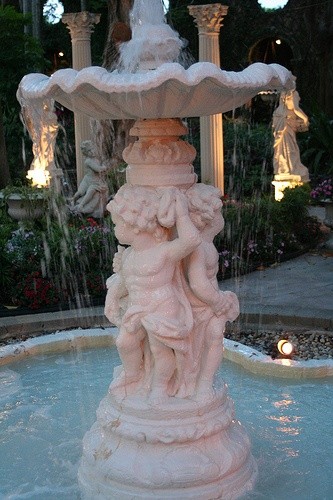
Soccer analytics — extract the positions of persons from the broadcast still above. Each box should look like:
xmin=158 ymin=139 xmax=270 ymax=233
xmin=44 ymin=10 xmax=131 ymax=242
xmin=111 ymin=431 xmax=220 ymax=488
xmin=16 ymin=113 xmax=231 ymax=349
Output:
xmin=68 ymin=140 xmax=106 ymax=211
xmin=184 ymin=183 xmax=241 ymax=402
xmin=271 ymin=90 xmax=310 ymax=177
xmin=103 ymin=189 xmax=201 ymax=408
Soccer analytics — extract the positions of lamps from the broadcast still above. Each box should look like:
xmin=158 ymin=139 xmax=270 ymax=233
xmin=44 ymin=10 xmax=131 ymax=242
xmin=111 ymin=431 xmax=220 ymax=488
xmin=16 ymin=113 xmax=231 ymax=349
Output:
xmin=262 ymin=339 xmax=293 ymax=360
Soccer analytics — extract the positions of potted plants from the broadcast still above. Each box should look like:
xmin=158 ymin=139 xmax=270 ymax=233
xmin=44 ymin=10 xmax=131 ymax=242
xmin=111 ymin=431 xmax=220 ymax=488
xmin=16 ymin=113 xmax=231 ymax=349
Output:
xmin=0 ymin=178 xmax=54 ymax=237
